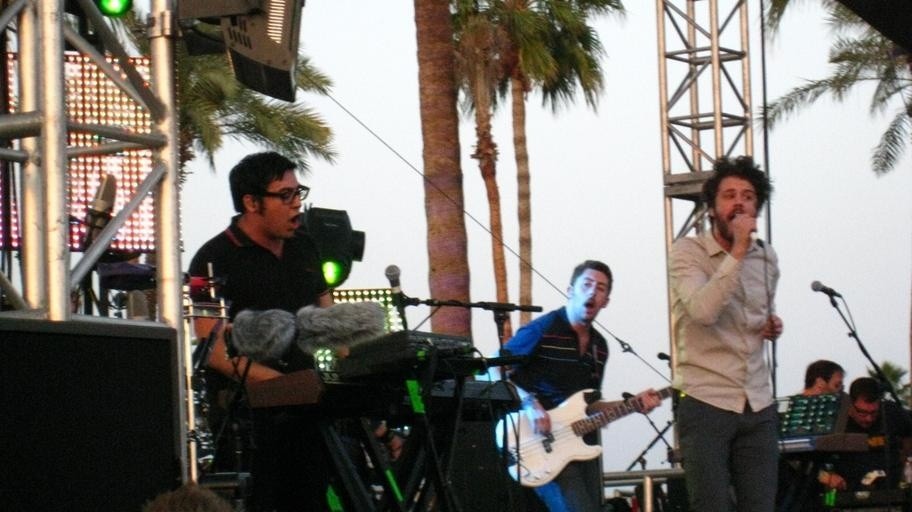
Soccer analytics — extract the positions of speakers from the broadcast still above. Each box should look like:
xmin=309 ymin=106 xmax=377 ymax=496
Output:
xmin=0 ymin=317 xmax=187 ymax=512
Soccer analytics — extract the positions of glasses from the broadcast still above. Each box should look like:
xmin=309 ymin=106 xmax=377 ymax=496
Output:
xmin=267 ymin=185 xmax=309 ymax=204
xmin=851 ymin=405 xmax=880 ymax=417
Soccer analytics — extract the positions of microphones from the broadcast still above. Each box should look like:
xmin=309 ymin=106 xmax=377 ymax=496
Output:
xmin=386 ymin=266 xmax=408 ymax=330
xmin=732 ymin=214 xmax=764 ymax=248
xmin=812 ymin=281 xmax=841 ymax=296
xmin=81 ymin=174 xmax=116 ymax=247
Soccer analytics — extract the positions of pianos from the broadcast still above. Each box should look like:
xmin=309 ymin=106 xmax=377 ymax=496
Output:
xmin=218 ymin=368 xmax=522 ymax=424
xmin=668 ymin=433 xmax=871 ymax=465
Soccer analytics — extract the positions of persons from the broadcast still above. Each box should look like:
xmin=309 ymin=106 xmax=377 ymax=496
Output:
xmin=188 ymin=152 xmax=402 ymax=512
xmin=667 ymin=156 xmax=783 ymax=512
xmin=788 ymin=360 xmax=910 ymax=512
xmin=498 ymin=260 xmax=662 ymax=512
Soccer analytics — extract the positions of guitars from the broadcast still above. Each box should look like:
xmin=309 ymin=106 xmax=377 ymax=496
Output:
xmin=495 ymin=386 xmax=673 ymax=488
